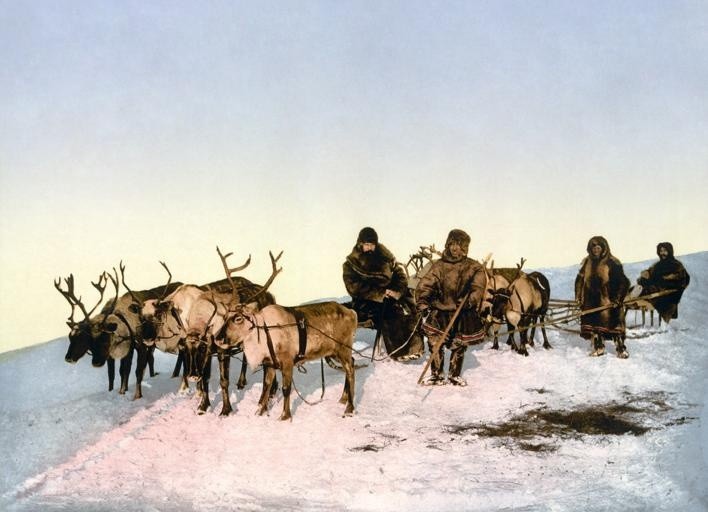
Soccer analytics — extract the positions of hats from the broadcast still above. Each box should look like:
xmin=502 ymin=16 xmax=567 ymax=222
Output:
xmin=446 ymin=229 xmax=469 ymax=255
xmin=356 ymin=227 xmax=377 ymax=251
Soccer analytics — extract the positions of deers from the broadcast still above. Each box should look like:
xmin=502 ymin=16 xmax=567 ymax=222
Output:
xmin=395 ymin=243 xmax=554 ymax=357
xmin=51 ymin=243 xmax=359 ymax=422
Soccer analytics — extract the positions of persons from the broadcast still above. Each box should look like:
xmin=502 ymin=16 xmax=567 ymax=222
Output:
xmin=576 ymin=236 xmax=691 ymax=358
xmin=344 ymin=228 xmax=488 ymax=387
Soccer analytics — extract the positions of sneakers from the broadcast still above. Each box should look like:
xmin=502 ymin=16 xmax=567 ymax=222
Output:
xmin=448 ymin=376 xmax=466 ymax=387
xmin=590 ymin=347 xmax=629 ymax=359
xmin=394 ymin=354 xmax=421 ymax=362
xmin=421 ymin=374 xmax=445 ymax=386
